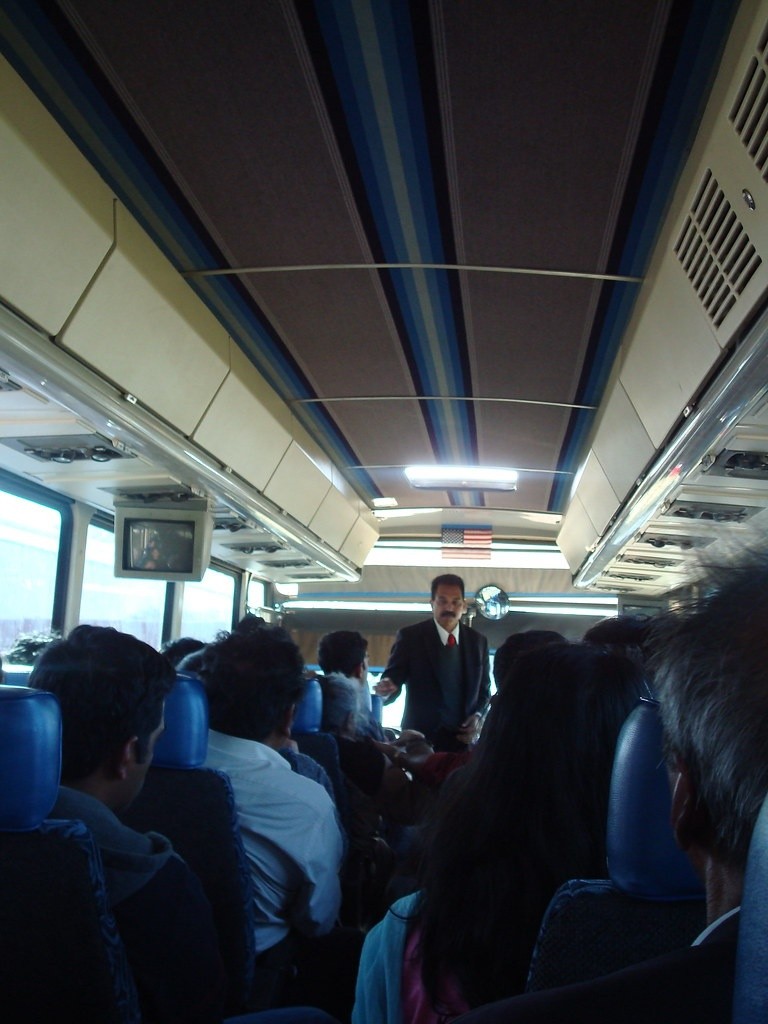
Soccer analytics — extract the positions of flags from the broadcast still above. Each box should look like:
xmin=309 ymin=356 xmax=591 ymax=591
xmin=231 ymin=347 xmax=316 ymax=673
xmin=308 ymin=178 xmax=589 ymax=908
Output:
xmin=442 ymin=527 xmax=493 ymax=559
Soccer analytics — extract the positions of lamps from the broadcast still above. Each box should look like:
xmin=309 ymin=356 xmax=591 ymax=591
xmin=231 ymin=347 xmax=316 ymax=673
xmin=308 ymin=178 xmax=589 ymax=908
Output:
xmin=405 ymin=466 xmax=519 ymax=491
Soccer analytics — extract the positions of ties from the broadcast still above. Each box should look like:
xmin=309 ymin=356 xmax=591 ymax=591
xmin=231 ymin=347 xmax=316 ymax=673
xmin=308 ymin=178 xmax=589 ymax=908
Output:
xmin=448 ymin=634 xmax=455 ymax=648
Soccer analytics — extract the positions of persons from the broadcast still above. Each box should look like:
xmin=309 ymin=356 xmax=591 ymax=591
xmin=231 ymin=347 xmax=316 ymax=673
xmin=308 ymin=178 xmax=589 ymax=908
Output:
xmin=0 ymin=545 xmax=768 ymax=1024
xmin=374 ymin=574 xmax=492 ymax=753
xmin=141 ymin=533 xmax=194 ymax=572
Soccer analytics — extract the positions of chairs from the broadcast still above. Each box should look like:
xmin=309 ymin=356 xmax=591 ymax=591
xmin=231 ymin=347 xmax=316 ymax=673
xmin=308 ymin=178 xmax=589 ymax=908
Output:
xmin=0 ymin=663 xmax=768 ymax=1024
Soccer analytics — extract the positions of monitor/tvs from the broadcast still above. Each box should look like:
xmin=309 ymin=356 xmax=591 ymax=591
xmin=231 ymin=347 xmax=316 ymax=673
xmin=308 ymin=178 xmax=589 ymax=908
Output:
xmin=113 ymin=506 xmax=214 ymax=582
xmin=617 ymin=598 xmax=669 ymax=618
